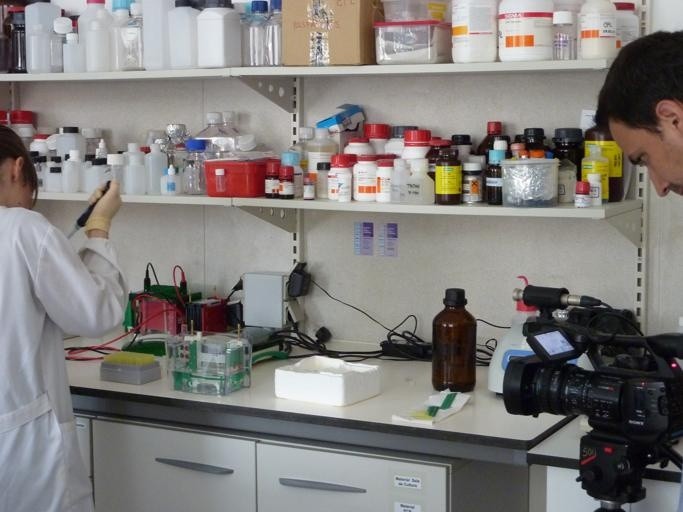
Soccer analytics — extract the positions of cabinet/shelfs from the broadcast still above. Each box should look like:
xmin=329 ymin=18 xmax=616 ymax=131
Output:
xmin=72 ymin=409 xmax=93 ymax=479
xmin=0 ymin=57 xmax=643 ymax=251
xmin=527 ymin=453 xmax=683 ymax=512
xmin=94 ymin=418 xmax=528 ymax=512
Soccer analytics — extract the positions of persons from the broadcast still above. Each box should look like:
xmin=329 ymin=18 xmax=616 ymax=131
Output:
xmin=593 ymin=30 xmax=682 ymax=200
xmin=0 ymin=124 xmax=128 ymax=511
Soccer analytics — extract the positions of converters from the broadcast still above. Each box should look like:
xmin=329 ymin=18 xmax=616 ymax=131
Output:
xmin=288 ymin=269 xmax=311 ymax=297
xmin=380 ymin=340 xmax=433 ymax=360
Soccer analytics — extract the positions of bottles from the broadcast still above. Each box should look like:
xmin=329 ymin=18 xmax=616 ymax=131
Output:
xmin=551 ymin=10 xmax=576 ymax=60
xmin=430 ymin=288 xmax=477 ymax=390
xmin=0 ymin=0 xmax=284 ymax=77
